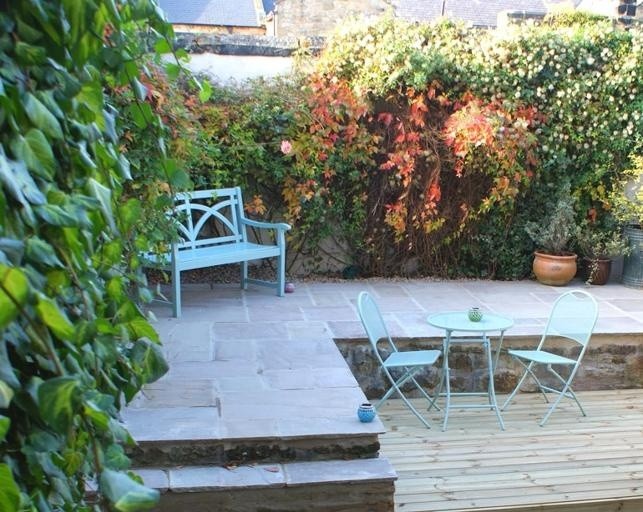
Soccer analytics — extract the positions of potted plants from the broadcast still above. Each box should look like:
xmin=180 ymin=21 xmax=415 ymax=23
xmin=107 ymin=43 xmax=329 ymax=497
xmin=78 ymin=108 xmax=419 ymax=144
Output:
xmin=527 ymin=156 xmax=643 ymax=289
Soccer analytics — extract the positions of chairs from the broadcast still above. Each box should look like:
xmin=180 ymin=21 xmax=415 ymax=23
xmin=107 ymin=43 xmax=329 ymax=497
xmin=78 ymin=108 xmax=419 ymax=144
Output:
xmin=355 ymin=286 xmax=599 ymax=432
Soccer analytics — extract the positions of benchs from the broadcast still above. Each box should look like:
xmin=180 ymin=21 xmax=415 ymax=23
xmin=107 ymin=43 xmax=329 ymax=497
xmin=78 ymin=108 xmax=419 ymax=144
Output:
xmin=133 ymin=192 xmax=291 ymax=318
